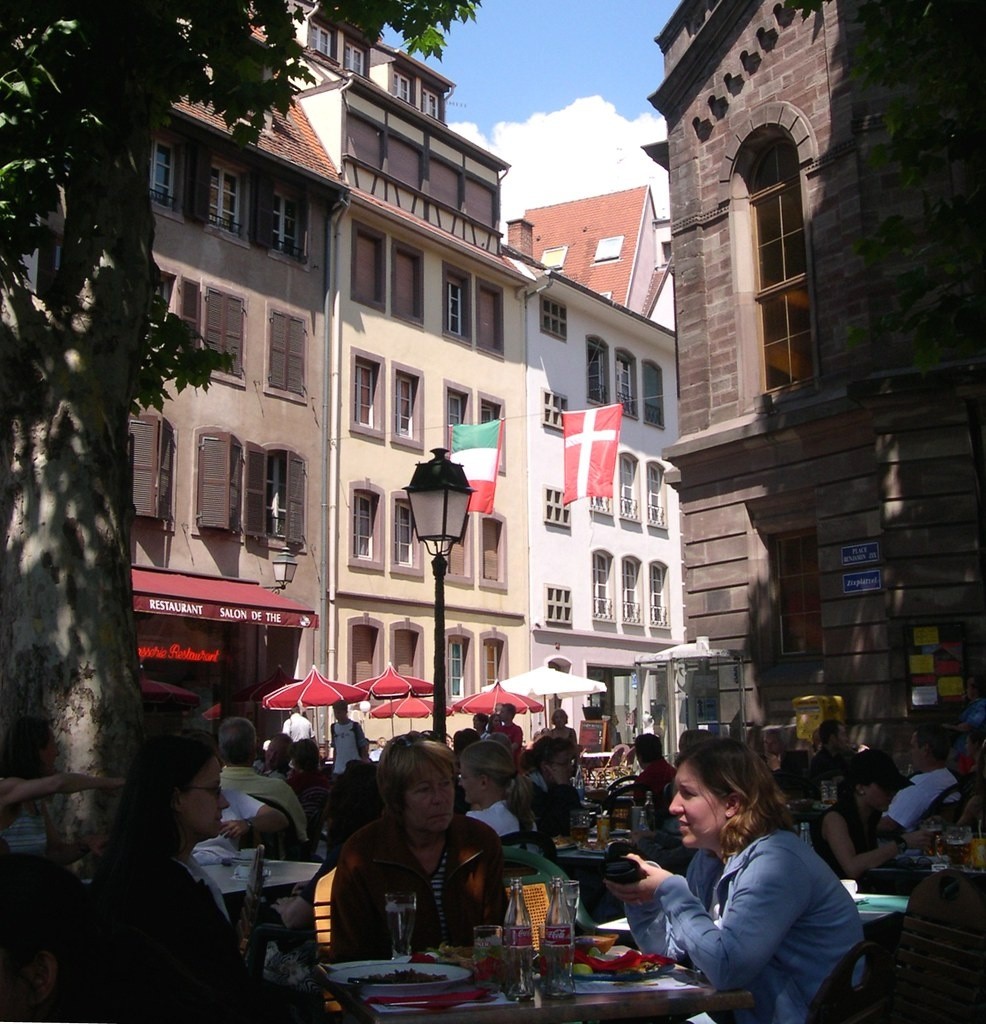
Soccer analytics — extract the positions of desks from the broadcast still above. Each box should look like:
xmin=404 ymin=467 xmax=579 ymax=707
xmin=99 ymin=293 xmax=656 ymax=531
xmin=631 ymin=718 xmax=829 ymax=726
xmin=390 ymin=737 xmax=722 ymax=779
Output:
xmin=204 ymin=859 xmax=323 ymax=914
xmin=556 ymin=836 xmax=626 ymax=920
xmin=849 ymin=891 xmax=911 ymax=956
xmin=869 ymin=864 xmax=986 ymax=895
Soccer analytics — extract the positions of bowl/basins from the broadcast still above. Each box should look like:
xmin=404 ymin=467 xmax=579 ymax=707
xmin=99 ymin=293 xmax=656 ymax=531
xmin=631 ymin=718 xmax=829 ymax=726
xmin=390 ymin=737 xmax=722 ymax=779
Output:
xmin=233 ymin=864 xmax=253 ymax=878
xmin=839 ymin=879 xmax=858 ymax=894
xmin=574 ymin=934 xmax=620 ymax=955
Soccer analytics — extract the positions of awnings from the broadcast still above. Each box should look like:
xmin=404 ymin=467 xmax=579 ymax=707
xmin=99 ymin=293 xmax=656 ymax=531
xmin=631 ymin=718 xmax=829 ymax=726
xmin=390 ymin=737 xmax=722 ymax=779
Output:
xmin=132 ymin=568 xmax=318 ymax=629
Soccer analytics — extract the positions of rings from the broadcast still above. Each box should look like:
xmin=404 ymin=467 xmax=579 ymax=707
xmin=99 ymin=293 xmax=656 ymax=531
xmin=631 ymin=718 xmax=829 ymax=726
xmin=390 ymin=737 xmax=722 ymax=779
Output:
xmin=636 ymin=898 xmax=643 ymax=907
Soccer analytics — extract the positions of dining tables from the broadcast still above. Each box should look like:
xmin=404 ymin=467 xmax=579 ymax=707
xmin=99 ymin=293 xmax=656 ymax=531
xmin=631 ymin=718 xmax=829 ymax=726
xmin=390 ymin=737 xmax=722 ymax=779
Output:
xmin=314 ymin=944 xmax=755 ymax=1024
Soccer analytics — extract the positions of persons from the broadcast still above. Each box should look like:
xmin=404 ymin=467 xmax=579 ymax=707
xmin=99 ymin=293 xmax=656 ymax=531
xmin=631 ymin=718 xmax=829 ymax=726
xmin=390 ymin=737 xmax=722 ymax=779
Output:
xmin=0 ymin=715 xmax=126 ymax=858
xmin=219 ymin=675 xmax=986 ymax=931
xmin=86 ymin=729 xmax=296 ymax=1024
xmin=602 ymin=738 xmax=866 ymax=1024
xmin=0 ymin=853 xmax=250 ymax=1024
xmin=330 ymin=731 xmax=507 ymax=964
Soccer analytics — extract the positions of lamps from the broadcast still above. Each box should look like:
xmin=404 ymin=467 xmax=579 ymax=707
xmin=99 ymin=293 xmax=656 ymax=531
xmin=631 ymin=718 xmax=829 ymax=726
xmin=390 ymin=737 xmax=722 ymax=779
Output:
xmin=258 ymin=546 xmax=298 ymax=593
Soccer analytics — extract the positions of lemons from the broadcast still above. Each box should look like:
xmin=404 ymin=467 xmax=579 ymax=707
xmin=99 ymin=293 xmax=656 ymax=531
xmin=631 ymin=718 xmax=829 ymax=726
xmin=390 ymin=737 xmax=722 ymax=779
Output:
xmin=572 ymin=964 xmax=594 ymax=975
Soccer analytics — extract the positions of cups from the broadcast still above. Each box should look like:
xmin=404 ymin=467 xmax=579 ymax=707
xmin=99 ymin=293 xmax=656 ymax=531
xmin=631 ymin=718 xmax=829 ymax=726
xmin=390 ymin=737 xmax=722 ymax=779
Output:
xmin=240 ymin=848 xmax=257 ymax=860
xmin=560 ymin=881 xmax=579 ymax=924
xmin=569 ymin=810 xmax=589 ymax=841
xmin=918 ymin=820 xmax=986 ymax=869
xmin=631 ymin=806 xmax=643 ymax=832
xmin=473 ymin=924 xmax=503 ymax=993
xmin=596 ymin=814 xmax=610 ymax=842
xmin=384 ymin=891 xmax=417 ymax=961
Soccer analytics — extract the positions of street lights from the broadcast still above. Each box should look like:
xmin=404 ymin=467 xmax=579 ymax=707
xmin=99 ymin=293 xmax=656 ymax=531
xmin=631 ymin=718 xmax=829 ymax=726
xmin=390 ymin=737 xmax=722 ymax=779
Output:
xmin=400 ymin=445 xmax=479 ymax=742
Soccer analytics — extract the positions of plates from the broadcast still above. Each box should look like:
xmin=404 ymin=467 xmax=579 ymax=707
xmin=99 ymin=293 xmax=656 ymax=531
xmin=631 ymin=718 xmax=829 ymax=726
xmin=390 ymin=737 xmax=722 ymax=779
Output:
xmin=573 ymin=963 xmax=674 ymax=980
xmin=549 ymin=828 xmax=632 ymax=853
xmin=321 ymin=960 xmax=408 ymax=974
xmin=325 ymin=963 xmax=473 ymax=998
xmin=233 ymin=859 xmax=255 ymax=864
xmin=230 ymin=876 xmax=268 ymax=883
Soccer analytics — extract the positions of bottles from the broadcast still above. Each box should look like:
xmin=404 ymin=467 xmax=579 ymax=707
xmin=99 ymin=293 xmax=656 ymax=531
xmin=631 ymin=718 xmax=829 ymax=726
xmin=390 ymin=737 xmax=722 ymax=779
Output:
xmin=503 ymin=876 xmax=536 ymax=999
xmin=637 ymin=811 xmax=648 ymax=831
xmin=544 ymin=877 xmax=576 ymax=998
xmin=799 ymin=822 xmax=814 ymax=850
xmin=847 ymin=743 xmax=869 ymax=752
xmin=575 ymin=766 xmax=585 ymax=800
xmin=644 ymin=791 xmax=655 ymax=830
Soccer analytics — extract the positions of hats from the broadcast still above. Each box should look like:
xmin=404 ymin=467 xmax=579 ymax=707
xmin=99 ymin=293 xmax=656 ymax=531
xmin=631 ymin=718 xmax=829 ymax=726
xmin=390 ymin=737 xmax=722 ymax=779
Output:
xmin=851 ymin=749 xmax=915 ymax=791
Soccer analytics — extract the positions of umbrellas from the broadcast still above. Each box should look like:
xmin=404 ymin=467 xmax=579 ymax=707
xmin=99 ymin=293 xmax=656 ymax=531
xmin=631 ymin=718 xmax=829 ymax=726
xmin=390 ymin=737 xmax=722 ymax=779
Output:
xmin=230 ymin=664 xmax=607 ymax=761
xmin=140 ymin=670 xmax=201 ymax=713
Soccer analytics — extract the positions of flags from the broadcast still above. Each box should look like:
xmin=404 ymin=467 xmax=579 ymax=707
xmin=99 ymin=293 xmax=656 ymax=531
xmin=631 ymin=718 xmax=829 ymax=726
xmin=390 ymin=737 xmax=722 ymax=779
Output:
xmin=562 ymin=403 xmax=624 ymax=507
xmin=448 ymin=418 xmax=505 ymax=516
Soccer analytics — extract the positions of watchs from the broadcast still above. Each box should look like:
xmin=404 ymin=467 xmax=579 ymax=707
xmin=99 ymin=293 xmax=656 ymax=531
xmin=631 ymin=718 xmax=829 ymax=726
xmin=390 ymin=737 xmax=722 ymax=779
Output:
xmin=895 ymin=837 xmax=908 ymax=855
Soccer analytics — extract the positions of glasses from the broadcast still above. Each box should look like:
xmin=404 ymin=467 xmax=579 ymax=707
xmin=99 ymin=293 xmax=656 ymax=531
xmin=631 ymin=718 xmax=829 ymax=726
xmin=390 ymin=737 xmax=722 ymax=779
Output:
xmin=186 ymin=784 xmax=223 ymax=798
xmin=387 ymin=729 xmax=442 ymax=766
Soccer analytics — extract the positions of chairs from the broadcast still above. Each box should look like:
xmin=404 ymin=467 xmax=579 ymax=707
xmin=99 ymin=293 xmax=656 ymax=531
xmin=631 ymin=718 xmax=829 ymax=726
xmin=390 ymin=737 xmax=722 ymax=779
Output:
xmin=236 ymin=744 xmax=986 ymax=1024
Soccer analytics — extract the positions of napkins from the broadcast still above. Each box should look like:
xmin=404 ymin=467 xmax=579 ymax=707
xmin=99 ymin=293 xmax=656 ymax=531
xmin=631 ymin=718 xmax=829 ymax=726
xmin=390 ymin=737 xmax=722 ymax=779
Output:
xmin=574 ymin=948 xmax=676 ymax=973
xmin=363 ymin=989 xmax=500 ymax=1011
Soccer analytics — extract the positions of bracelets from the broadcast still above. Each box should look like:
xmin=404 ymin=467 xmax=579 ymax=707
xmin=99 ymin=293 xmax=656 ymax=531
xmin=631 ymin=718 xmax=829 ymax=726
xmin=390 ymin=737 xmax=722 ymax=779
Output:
xmin=245 ymin=819 xmax=253 ymax=833
